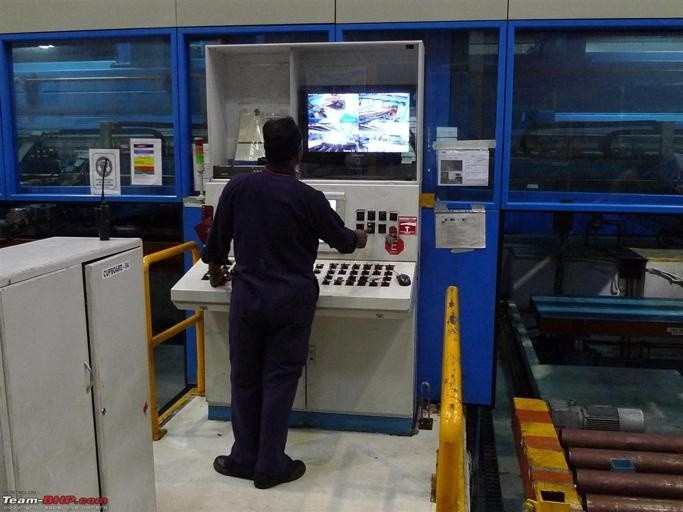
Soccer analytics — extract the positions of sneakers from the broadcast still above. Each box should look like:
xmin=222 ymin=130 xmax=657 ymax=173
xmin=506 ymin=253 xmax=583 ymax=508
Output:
xmin=214 ymin=455 xmax=255 ymax=479
xmin=254 ymin=460 xmax=305 ymax=489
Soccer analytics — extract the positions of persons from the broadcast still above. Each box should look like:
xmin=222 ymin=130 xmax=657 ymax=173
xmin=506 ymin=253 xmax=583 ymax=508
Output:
xmin=202 ymin=114 xmax=368 ymax=490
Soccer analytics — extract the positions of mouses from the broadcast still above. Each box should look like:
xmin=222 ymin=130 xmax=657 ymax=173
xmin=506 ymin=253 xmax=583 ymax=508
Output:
xmin=399 ymin=274 xmax=410 ymax=285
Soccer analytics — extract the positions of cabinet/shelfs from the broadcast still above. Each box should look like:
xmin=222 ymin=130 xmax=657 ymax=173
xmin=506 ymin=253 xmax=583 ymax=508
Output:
xmin=203 ymin=310 xmax=417 ymax=435
xmin=0 ymin=235 xmax=158 ymax=512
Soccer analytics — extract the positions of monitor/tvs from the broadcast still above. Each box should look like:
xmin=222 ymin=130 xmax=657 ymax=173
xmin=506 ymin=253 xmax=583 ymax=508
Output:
xmin=318 ymin=198 xmax=337 ymax=242
xmin=300 ymin=84 xmax=417 ymax=181
